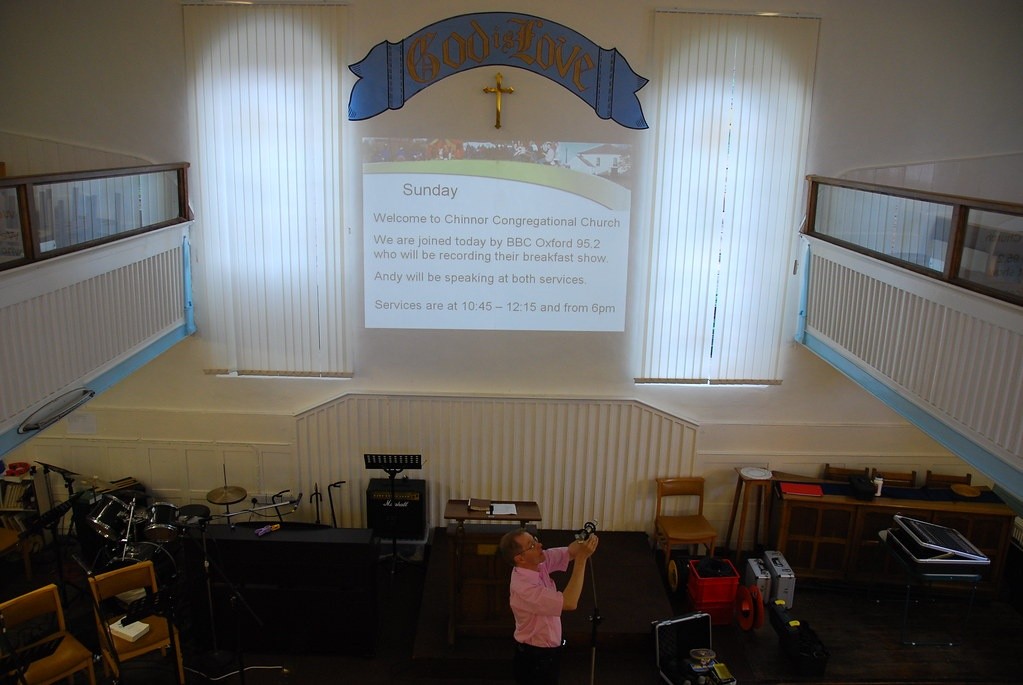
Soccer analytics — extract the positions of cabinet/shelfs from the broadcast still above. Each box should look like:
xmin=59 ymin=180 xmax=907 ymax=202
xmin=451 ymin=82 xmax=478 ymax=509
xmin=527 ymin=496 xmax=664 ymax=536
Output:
xmin=0 ymin=463 xmax=54 ymax=558
xmin=769 ymin=473 xmax=1017 ymax=610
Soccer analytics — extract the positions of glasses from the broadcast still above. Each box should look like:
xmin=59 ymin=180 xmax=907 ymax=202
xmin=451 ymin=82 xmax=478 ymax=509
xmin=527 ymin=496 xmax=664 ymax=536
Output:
xmin=514 ymin=536 xmax=538 ymax=556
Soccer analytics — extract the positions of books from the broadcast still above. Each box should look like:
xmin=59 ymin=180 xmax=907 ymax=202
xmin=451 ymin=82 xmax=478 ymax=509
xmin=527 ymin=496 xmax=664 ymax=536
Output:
xmin=108 ymin=615 xmax=150 ymax=642
xmin=468 ymin=498 xmax=491 ymax=511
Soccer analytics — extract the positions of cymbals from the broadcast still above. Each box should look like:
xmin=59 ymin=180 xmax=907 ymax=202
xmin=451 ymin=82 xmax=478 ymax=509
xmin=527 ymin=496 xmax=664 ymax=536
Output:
xmin=78 ymin=478 xmax=119 ymax=490
xmin=206 ymin=485 xmax=247 ymax=506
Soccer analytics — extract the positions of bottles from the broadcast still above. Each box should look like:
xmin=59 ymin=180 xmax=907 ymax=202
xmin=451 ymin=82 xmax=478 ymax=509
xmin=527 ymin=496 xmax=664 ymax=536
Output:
xmin=874 ymin=473 xmax=883 ymax=496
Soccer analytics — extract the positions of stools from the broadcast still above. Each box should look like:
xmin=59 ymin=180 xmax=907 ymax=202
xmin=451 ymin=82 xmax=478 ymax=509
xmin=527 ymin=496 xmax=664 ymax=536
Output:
xmin=727 ymin=463 xmax=774 ymax=555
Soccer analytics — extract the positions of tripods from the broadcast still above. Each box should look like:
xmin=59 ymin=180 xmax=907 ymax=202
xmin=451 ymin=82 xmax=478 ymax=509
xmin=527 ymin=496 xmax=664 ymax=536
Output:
xmin=364 ymin=454 xmax=428 ymax=596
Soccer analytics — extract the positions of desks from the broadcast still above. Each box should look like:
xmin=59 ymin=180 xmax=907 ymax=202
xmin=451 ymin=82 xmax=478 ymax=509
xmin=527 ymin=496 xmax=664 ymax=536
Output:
xmin=857 ymin=526 xmax=987 ymax=651
xmin=181 ymin=519 xmax=386 ymax=661
xmin=0 ymin=525 xmax=35 ymax=579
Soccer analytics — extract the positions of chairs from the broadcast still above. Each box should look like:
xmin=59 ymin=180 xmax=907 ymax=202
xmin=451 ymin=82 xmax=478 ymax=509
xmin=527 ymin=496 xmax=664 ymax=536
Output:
xmin=86 ymin=561 xmax=188 ymax=684
xmin=823 ymin=461 xmax=972 ymax=487
xmin=653 ymin=476 xmax=722 ymax=565
xmin=0 ymin=582 xmax=99 ymax=685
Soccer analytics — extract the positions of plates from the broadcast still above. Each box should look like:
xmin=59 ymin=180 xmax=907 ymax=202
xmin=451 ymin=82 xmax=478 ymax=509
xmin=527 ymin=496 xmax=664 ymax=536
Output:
xmin=740 ymin=466 xmax=773 ymax=480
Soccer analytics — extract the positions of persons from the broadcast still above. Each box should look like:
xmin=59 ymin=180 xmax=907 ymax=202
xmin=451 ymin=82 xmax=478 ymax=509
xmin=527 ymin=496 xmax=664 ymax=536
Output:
xmin=500 ymin=529 xmax=599 ymax=685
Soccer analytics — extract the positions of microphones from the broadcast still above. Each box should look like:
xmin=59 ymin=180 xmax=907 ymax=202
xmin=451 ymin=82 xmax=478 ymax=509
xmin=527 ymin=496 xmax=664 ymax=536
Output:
xmin=70 ymin=554 xmax=93 ymax=577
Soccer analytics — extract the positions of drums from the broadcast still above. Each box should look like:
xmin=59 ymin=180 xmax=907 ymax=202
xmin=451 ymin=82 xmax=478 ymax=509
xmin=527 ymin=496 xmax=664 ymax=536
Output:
xmin=145 ymin=500 xmax=179 ymax=542
xmin=123 ymin=505 xmax=148 ymax=540
xmin=85 ymin=493 xmax=137 ymax=540
xmin=112 ymin=542 xmax=178 ymax=598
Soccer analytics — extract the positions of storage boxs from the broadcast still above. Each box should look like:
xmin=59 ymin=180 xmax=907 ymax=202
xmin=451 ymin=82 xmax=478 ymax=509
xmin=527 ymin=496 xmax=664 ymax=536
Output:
xmin=686 ymin=556 xmax=742 ymax=624
xmin=766 ymin=605 xmax=833 ymax=676
xmin=647 ymin=608 xmax=740 ymax=685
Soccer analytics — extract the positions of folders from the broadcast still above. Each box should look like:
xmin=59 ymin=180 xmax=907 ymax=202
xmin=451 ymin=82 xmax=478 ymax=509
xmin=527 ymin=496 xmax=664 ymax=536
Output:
xmin=780 ymin=482 xmax=824 ymax=497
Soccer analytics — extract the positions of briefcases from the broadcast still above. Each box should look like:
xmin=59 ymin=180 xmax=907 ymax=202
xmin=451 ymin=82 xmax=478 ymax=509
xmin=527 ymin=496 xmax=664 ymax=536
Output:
xmin=885 ymin=515 xmax=990 ymax=575
xmin=745 ymin=558 xmax=771 ymax=604
xmin=648 ymin=610 xmax=737 ymax=685
xmin=765 ymin=551 xmax=796 ymax=609
